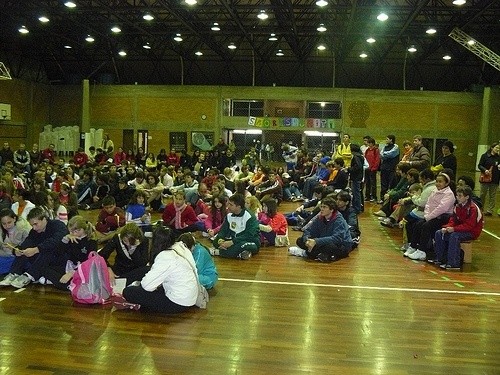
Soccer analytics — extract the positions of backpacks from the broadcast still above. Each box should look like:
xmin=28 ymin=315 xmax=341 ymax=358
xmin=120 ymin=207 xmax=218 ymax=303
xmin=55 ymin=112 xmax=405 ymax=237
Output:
xmin=67 ymin=251 xmax=115 ymax=305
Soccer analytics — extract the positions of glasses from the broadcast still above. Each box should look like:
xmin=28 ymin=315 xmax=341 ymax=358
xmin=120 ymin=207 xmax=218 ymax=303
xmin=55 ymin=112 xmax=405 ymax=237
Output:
xmin=320 ymin=205 xmax=328 ymax=208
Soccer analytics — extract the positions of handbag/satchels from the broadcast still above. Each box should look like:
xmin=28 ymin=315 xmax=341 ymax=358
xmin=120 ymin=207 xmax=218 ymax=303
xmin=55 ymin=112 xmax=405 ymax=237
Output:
xmin=196 ymin=282 xmax=210 ymax=309
xmin=273 ymin=235 xmax=290 ymax=247
xmin=479 ymin=166 xmax=493 ymax=183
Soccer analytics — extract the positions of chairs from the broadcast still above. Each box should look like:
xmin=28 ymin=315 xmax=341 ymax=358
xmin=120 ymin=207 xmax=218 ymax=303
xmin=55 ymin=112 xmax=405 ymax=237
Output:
xmin=85 ymin=128 xmax=104 ymax=155
xmin=38 ymin=124 xmax=79 ymax=157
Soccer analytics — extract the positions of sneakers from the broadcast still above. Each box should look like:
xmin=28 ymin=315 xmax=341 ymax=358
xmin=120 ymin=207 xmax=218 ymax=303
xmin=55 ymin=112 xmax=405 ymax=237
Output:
xmin=317 ymin=252 xmax=334 ymax=262
xmin=403 ymin=246 xmax=417 ymax=257
xmin=408 ymin=249 xmax=427 ymax=260
xmin=0 ymin=272 xmax=21 ymax=286
xmin=10 ymin=274 xmax=33 ymax=288
xmin=287 ymin=246 xmax=308 ymax=257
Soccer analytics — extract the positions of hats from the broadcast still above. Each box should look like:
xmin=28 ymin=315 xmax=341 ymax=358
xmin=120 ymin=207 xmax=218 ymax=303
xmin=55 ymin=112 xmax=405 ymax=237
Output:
xmin=320 ymin=156 xmax=330 ymax=164
xmin=282 ymin=172 xmax=291 ymax=178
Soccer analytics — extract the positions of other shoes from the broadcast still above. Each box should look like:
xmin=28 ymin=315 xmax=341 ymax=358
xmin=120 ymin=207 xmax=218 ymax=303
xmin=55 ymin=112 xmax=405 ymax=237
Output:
xmin=83 ymin=204 xmax=90 ymax=211
xmin=291 ymin=198 xmax=296 ymax=203
xmin=381 ymin=218 xmax=394 ymax=227
xmin=483 ymin=212 xmax=491 ymax=216
xmin=239 ymin=250 xmax=252 ymax=259
xmin=297 ymin=196 xmax=304 ymax=201
xmin=373 ymin=210 xmax=386 ymax=217
xmin=427 ymin=257 xmax=442 ymax=264
xmin=208 ymin=247 xmax=217 ymax=256
xmin=379 ymin=216 xmax=386 ymax=220
xmin=400 ymin=243 xmax=410 ymax=250
xmin=376 ymin=200 xmax=383 ymax=204
xmin=439 ymin=263 xmax=460 ymax=270
xmin=363 ymin=196 xmax=370 ymax=202
xmin=370 ymin=197 xmax=376 ymax=202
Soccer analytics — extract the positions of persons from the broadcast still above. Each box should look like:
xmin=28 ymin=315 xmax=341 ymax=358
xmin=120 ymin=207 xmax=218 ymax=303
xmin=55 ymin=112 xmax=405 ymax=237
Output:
xmin=404 ymin=168 xmax=456 ymax=261
xmin=428 ymin=184 xmax=483 ymax=269
xmin=0 ymin=133 xmax=500 ymax=315
